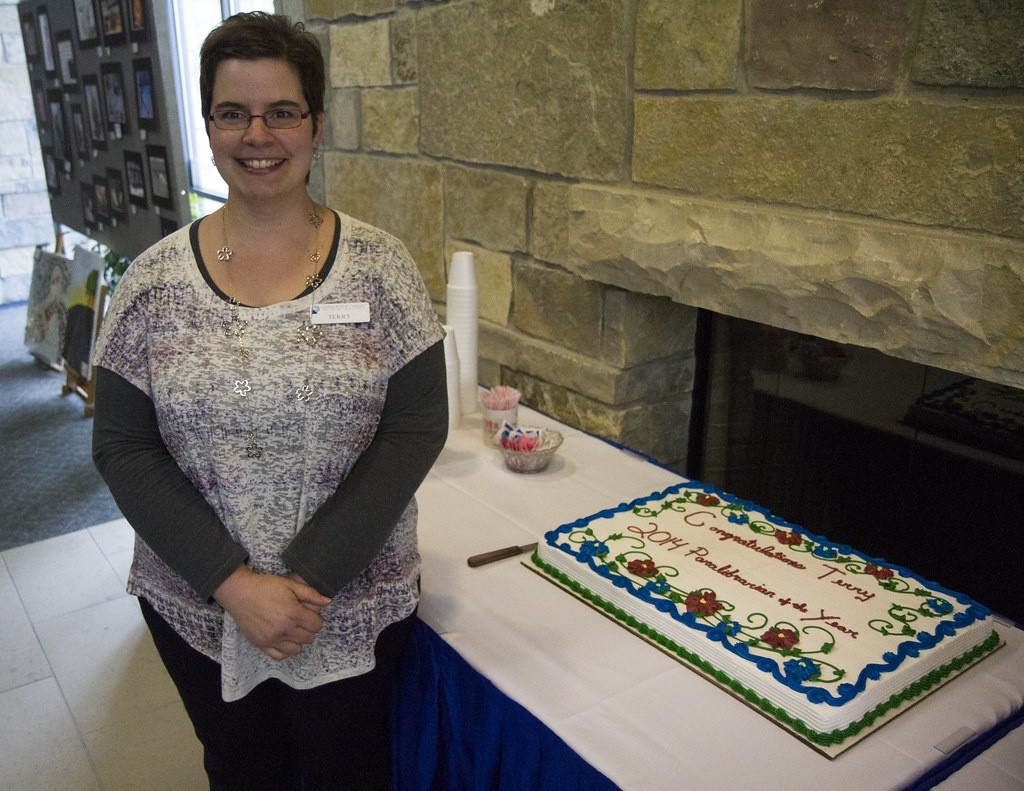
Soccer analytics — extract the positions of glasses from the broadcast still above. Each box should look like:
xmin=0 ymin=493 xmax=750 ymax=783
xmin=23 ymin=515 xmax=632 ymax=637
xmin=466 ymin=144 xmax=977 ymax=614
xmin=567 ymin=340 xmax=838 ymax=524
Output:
xmin=208 ymin=109 xmax=312 ymax=130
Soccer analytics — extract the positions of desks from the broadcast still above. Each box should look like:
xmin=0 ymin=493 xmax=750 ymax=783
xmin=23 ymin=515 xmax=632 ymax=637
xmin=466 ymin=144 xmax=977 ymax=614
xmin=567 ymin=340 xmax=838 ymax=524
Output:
xmin=392 ymin=385 xmax=1024 ymax=791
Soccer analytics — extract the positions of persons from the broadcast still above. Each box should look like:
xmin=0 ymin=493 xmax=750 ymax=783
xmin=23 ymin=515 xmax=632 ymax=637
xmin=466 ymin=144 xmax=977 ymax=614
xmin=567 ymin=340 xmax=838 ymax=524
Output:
xmin=91 ymin=11 xmax=449 ymax=791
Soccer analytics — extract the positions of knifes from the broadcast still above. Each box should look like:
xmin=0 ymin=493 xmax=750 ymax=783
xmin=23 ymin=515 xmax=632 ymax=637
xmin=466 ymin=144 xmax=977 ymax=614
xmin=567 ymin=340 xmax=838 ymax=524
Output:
xmin=468 ymin=543 xmax=538 ymax=567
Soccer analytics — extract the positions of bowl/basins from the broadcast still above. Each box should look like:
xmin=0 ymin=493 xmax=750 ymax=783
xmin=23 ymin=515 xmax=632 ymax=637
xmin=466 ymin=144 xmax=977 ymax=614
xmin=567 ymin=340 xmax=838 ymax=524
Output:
xmin=494 ymin=426 xmax=564 ymax=473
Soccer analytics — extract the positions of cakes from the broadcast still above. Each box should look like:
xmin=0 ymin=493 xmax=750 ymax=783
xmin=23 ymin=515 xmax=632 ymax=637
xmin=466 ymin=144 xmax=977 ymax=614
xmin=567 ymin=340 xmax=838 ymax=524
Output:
xmin=530 ymin=478 xmax=1001 ymax=747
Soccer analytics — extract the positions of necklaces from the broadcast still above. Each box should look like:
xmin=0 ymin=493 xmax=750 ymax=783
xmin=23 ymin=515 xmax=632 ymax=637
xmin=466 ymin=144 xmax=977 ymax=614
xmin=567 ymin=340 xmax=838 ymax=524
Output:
xmin=217 ymin=193 xmax=325 ymax=458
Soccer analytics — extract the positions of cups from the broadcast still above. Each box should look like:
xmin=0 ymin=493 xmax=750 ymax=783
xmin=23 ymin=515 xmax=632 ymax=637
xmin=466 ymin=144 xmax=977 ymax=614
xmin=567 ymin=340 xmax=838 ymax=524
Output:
xmin=483 ymin=406 xmax=518 ymax=449
xmin=442 ymin=251 xmax=478 ymax=429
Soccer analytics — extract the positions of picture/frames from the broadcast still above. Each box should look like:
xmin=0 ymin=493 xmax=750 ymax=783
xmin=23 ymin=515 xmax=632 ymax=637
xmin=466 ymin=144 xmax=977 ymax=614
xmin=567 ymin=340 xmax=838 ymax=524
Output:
xmin=20 ymin=1 xmax=178 ymax=242
